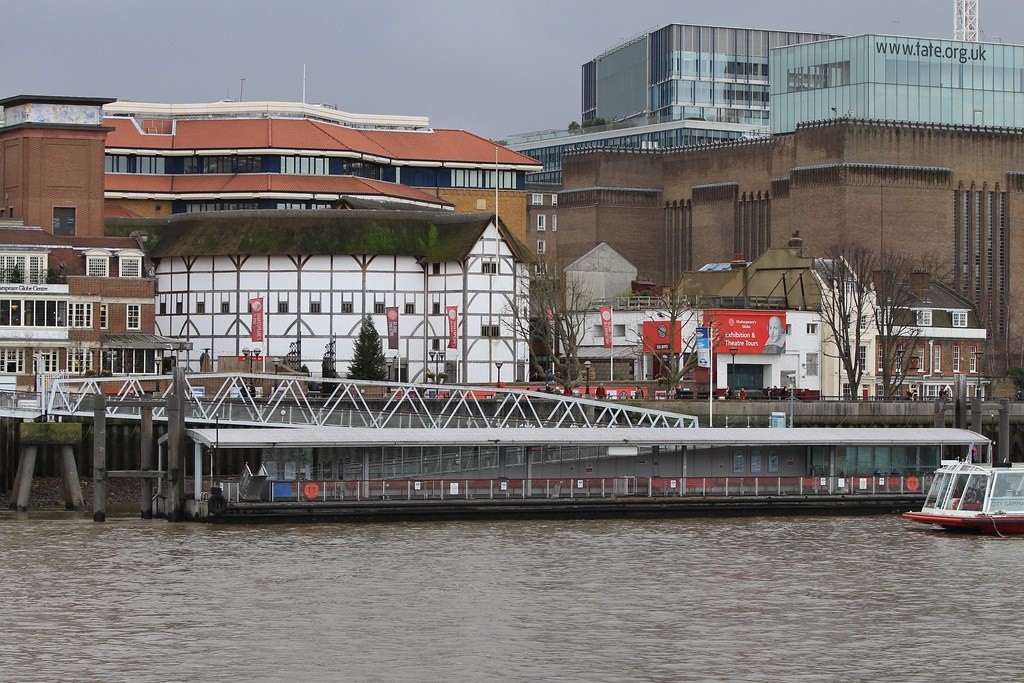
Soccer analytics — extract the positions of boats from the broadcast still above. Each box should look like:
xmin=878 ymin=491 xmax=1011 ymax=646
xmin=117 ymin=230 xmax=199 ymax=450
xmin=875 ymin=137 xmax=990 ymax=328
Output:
xmin=903 ymin=461 xmax=1024 ymax=535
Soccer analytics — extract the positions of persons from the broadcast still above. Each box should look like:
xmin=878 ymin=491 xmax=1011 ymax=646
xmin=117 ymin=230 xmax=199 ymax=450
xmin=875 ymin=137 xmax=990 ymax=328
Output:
xmin=725 ymin=384 xmax=806 ymax=400
xmin=240 ymin=382 xmax=256 ymax=397
xmin=905 ymin=388 xmax=1023 ymax=402
xmin=635 ymin=386 xmax=643 ymax=399
xmin=668 ymin=385 xmax=681 ymax=400
xmin=596 ymin=382 xmax=606 ymax=399
xmin=526 ymin=383 xmax=573 ymax=397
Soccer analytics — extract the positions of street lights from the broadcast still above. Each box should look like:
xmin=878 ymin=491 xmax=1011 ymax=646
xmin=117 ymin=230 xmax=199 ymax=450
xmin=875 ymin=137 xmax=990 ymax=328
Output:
xmin=584 ymin=360 xmax=592 ymax=394
xmin=974 ymin=350 xmax=984 ymax=400
xmin=495 ymin=360 xmax=503 ymax=388
xmin=660 ymin=354 xmax=676 ymax=398
xmin=729 ymin=347 xmax=738 ymax=394
xmin=272 ymin=357 xmax=280 ymax=375
xmin=428 ymin=349 xmax=445 ymax=384
xmin=242 ymin=347 xmax=262 ymax=374
